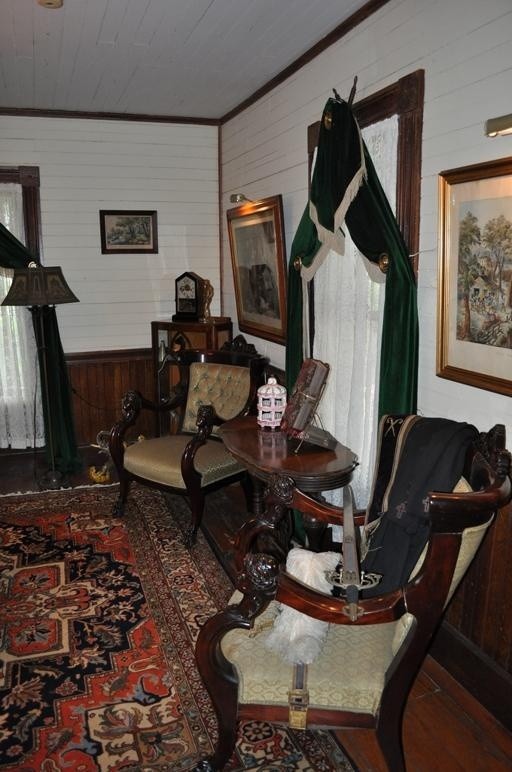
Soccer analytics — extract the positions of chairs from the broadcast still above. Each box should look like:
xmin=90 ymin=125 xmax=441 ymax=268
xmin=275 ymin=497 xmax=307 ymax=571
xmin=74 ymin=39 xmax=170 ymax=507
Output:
xmin=108 ymin=334 xmax=271 ymax=550
xmin=192 ymin=413 xmax=512 ymax=772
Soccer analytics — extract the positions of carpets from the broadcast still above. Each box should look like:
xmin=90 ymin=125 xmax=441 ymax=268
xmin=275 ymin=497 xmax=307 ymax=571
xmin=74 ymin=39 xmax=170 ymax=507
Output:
xmin=0 ymin=479 xmax=362 ymax=772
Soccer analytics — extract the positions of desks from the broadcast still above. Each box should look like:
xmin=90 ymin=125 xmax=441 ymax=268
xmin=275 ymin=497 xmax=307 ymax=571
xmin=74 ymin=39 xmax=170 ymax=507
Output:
xmin=220 ymin=414 xmax=359 ymax=564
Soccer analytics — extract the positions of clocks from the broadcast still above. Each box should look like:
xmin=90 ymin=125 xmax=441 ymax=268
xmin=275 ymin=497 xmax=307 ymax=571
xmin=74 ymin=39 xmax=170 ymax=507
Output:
xmin=173 ymin=271 xmax=214 ymax=323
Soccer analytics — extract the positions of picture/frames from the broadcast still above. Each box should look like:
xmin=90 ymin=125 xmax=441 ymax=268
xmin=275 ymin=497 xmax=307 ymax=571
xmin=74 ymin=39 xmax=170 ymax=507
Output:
xmin=226 ymin=193 xmax=288 ymax=347
xmin=434 ymin=156 xmax=512 ymax=397
xmin=97 ymin=208 xmax=159 ymax=254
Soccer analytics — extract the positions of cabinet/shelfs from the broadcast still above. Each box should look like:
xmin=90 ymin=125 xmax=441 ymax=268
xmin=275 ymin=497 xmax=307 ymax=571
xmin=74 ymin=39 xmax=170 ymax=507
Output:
xmin=149 ymin=320 xmax=233 ymax=436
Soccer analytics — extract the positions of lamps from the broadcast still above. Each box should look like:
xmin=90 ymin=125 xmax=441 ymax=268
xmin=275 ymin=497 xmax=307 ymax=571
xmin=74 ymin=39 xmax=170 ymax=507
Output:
xmin=0 ymin=252 xmax=80 ymax=488
xmin=230 ymin=190 xmax=253 ymax=204
